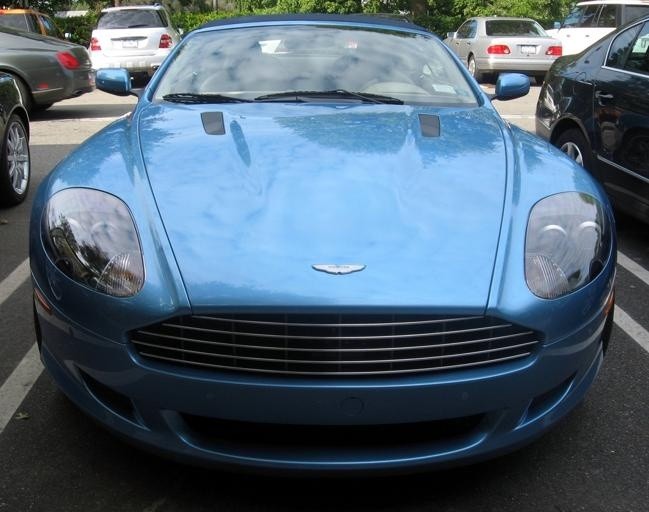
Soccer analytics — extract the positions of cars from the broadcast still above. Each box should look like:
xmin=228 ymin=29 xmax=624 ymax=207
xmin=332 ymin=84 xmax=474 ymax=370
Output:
xmin=26 ymin=10 xmax=621 ymax=494
xmin=0 ymin=22 xmax=98 ymax=122
xmin=441 ymin=15 xmax=566 ymax=88
xmin=0 ymin=68 xmax=36 ymax=212
xmin=531 ymin=12 xmax=649 ymax=236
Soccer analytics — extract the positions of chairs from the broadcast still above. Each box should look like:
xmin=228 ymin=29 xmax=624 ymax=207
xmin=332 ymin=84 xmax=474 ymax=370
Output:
xmin=198 ymin=35 xmax=417 ymax=96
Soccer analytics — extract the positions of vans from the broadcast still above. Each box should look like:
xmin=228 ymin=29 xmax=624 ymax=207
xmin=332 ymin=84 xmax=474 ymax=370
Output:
xmin=554 ymin=1 xmax=649 ymax=59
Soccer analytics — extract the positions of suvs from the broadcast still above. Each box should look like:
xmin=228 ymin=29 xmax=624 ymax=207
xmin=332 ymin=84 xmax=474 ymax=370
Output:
xmin=86 ymin=3 xmax=189 ymax=87
xmin=1 ymin=9 xmax=71 ymax=42
